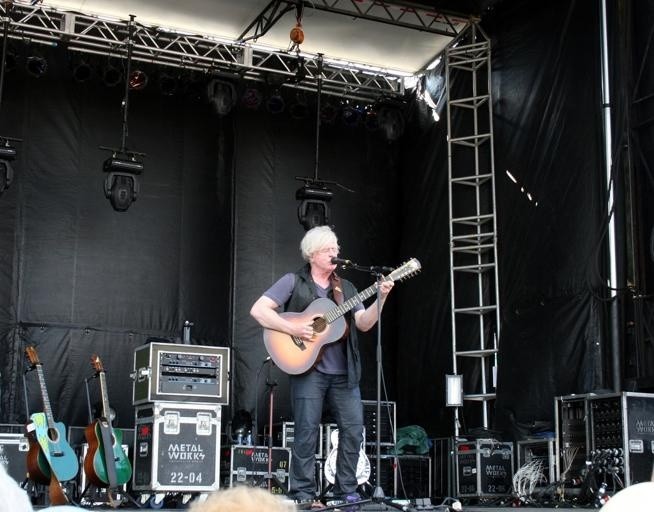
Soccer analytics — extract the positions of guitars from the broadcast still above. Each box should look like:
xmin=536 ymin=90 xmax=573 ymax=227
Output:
xmin=324 ymin=429 xmax=371 ymax=486
xmin=84 ymin=353 xmax=132 ymax=487
xmin=263 ymin=258 xmax=421 ymax=376
xmin=25 ymin=346 xmax=79 ymax=485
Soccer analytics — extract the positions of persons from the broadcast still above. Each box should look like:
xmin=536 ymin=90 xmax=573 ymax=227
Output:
xmin=248 ymin=224 xmax=395 ymax=511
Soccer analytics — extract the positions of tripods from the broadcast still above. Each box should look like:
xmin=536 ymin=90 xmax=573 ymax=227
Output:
xmin=312 ymin=263 xmax=419 ymax=511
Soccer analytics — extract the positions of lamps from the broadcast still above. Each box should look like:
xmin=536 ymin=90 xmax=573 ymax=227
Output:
xmin=95 ymin=12 xmax=149 ymax=216
xmin=204 ymin=77 xmax=239 ymax=116
xmin=294 ymin=52 xmax=336 ymax=233
xmin=2 ymin=4 xmax=25 ymax=193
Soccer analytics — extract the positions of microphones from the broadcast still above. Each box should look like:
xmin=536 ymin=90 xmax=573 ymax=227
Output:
xmin=331 ymin=256 xmax=354 ymax=268
